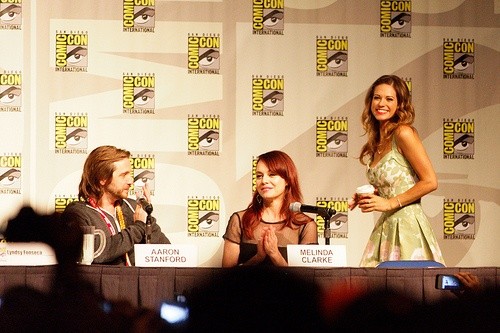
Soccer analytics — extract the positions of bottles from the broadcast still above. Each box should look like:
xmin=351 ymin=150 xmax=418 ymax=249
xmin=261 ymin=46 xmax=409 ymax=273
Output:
xmin=174 ymin=296 xmax=188 ymax=321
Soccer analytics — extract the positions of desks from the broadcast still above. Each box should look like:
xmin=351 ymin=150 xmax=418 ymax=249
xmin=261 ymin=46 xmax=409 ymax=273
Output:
xmin=0 ymin=265 xmax=500 ymax=333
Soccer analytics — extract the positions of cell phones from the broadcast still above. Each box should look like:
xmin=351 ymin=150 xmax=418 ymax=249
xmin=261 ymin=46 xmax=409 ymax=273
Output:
xmin=176 ymin=295 xmax=187 ymax=303
xmin=159 ymin=301 xmax=190 ymax=324
xmin=103 ymin=303 xmax=112 ymax=313
xmin=435 ymin=274 xmax=465 ymax=290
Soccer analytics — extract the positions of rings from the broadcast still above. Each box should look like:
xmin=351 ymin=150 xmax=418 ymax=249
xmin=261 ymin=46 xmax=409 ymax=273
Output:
xmin=368 ymin=200 xmax=371 ymax=204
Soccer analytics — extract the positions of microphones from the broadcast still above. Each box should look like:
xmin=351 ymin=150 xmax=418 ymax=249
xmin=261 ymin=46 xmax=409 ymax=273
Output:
xmin=136 ymin=195 xmax=153 ymax=213
xmin=288 ymin=201 xmax=336 ymax=215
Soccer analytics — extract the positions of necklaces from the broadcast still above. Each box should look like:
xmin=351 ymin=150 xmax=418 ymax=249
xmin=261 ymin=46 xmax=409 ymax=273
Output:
xmin=375 ymin=147 xmax=386 ymax=155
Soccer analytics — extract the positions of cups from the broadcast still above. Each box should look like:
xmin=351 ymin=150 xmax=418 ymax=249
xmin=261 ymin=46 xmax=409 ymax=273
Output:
xmin=77 ymin=226 xmax=107 ymax=265
xmin=356 ymin=185 xmax=375 ymax=213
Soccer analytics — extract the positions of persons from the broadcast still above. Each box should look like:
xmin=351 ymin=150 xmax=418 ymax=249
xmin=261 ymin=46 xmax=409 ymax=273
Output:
xmin=450 ymin=272 xmax=480 ymax=296
xmin=60 ymin=144 xmax=171 ymax=265
xmin=222 ymin=150 xmax=318 ymax=267
xmin=347 ymin=74 xmax=446 ymax=267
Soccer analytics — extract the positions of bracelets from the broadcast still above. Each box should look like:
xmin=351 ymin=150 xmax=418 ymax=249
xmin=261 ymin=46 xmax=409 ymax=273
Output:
xmin=395 ymin=195 xmax=403 ymax=207
